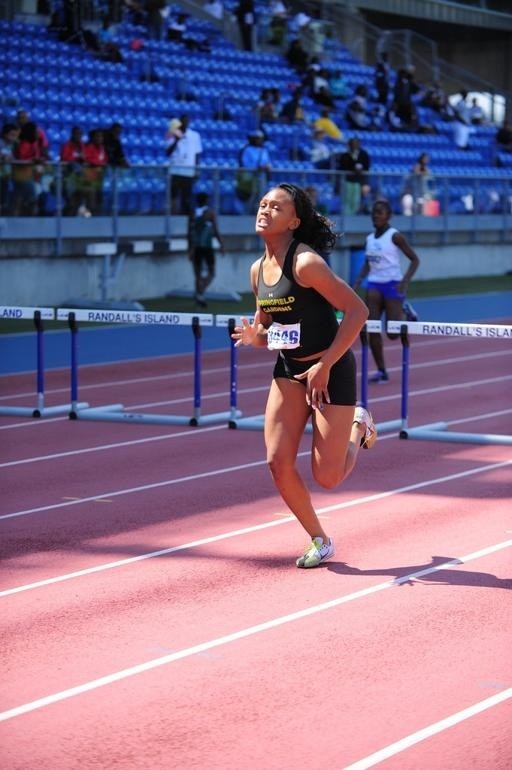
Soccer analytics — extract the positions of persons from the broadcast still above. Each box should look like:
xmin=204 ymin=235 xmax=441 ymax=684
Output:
xmin=185 ymin=190 xmax=228 ymax=313
xmin=238 ymin=87 xmax=374 ymax=211
xmin=1 ymin=109 xmax=128 ymax=218
xmin=352 ymin=200 xmax=419 ymax=386
xmin=415 ymin=153 xmax=431 ymax=174
xmin=1 ymin=1 xmax=301 ymax=60
xmin=230 ymin=181 xmax=379 ymax=570
xmin=167 ymin=114 xmax=203 ymax=213
xmin=284 ymin=39 xmax=512 ymax=153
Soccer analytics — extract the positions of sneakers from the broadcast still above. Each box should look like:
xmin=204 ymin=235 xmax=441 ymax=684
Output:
xmin=194 ymin=296 xmax=207 ymax=308
xmin=404 ymin=302 xmax=418 ymax=320
xmin=352 ymin=404 xmax=376 ymax=450
xmin=79 ymin=206 xmax=91 ymax=216
xmin=366 ymin=372 xmax=390 ymax=386
xmin=297 ymin=537 xmax=337 ymax=568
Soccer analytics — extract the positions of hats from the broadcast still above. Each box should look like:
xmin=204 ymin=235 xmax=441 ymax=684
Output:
xmin=248 ymin=129 xmax=264 ymax=139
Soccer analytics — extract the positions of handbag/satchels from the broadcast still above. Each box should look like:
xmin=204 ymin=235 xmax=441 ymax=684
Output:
xmin=236 ymin=179 xmax=253 ymax=201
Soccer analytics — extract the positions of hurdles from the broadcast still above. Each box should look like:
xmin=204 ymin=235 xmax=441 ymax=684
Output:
xmin=0 ymin=305 xmax=512 ymax=445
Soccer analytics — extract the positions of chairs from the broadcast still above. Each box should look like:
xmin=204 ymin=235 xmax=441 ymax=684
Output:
xmin=0 ymin=0 xmax=512 ymax=214
